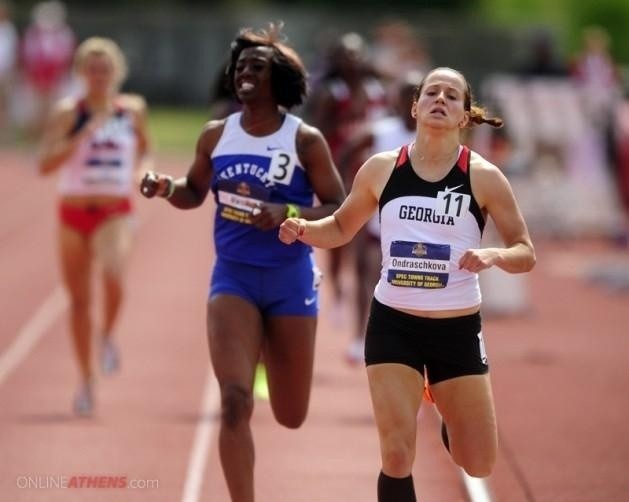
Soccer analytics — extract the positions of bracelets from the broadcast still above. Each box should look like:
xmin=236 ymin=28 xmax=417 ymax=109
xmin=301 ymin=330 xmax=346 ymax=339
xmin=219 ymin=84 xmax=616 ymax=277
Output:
xmin=286 ymin=204 xmax=299 ymax=219
xmin=163 ymin=174 xmax=176 ymax=201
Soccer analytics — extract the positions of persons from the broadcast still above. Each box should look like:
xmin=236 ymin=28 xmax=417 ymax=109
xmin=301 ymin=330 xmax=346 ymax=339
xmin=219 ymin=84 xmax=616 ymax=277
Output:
xmin=0 ymin=1 xmax=629 ymax=365
xmin=36 ymin=35 xmax=152 ymax=417
xmin=138 ymin=30 xmax=347 ymax=501
xmin=276 ymin=65 xmax=537 ymax=502
